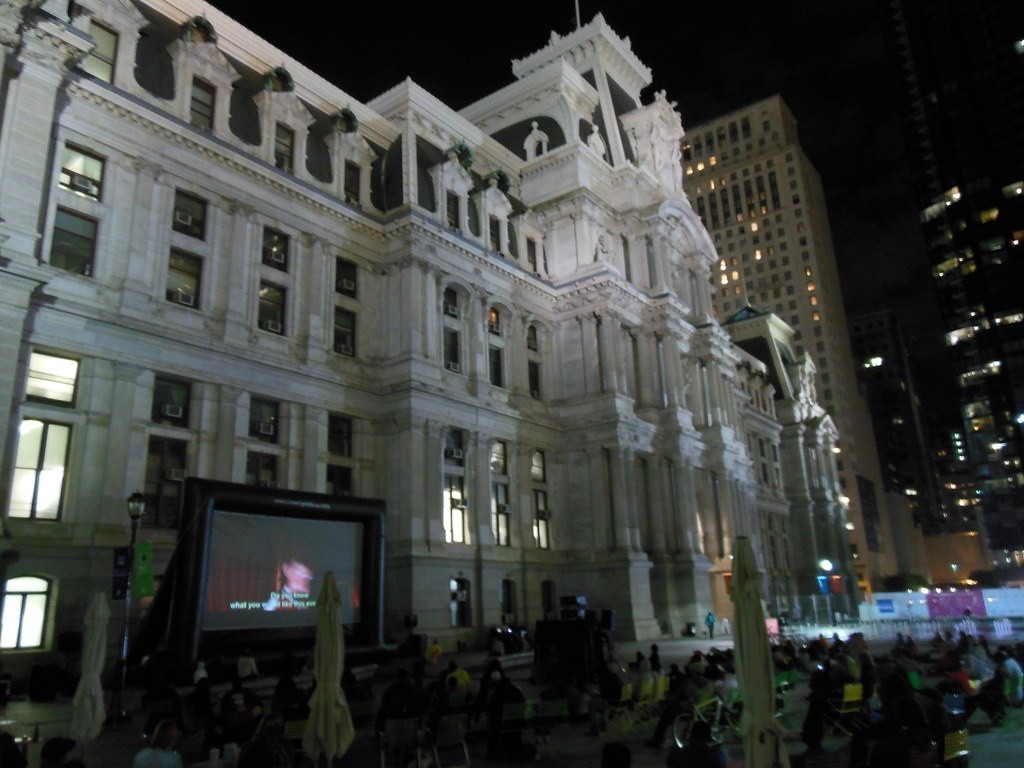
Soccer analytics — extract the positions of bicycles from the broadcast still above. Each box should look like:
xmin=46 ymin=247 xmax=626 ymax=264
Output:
xmin=672 ymin=676 xmax=784 ymax=754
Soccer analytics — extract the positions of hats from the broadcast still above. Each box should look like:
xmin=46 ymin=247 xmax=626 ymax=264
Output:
xmin=693 ymin=650 xmax=703 ymax=658
xmin=41 ymin=737 xmax=76 ymax=758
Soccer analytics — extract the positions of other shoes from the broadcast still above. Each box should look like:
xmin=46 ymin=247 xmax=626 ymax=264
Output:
xmin=376 ymin=730 xmax=384 ymax=745
xmin=584 ymin=731 xmax=599 ymax=737
xmin=182 ymin=728 xmax=199 ymax=736
xmin=645 ymin=740 xmax=660 ymax=749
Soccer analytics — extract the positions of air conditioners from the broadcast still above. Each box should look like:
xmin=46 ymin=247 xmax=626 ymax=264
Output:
xmin=254 ymin=422 xmax=274 ymax=436
xmin=453 ymin=498 xmax=467 ymax=508
xmin=340 ymin=278 xmax=355 ymax=291
xmin=263 ymin=319 xmax=283 ymax=332
xmin=340 ymin=343 xmax=353 ymax=355
xmin=540 ymin=510 xmax=551 ymax=519
xmin=447 ymin=362 xmax=461 ymax=372
xmin=445 ymin=304 xmax=460 ymax=315
xmin=162 ymin=405 xmax=183 ymax=418
xmin=262 ymin=481 xmax=280 ymax=488
xmin=71 ymin=174 xmax=92 ymax=191
xmin=165 ymin=469 xmax=189 ymax=482
xmin=175 ymin=210 xmax=193 ymax=226
xmin=269 ymin=251 xmax=286 ymax=263
xmin=489 ymin=324 xmax=502 ymax=333
xmin=446 ymin=448 xmax=462 ymax=459
xmin=174 ymin=292 xmax=195 ymax=306
xmin=500 ymin=504 xmax=512 ymax=514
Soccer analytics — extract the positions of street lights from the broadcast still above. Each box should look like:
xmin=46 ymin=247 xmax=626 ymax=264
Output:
xmin=104 ymin=490 xmax=146 ymax=726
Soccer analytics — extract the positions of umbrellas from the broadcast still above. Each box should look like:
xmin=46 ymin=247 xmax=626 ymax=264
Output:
xmin=70 ymin=592 xmax=111 ymax=766
xmin=302 ymin=569 xmax=354 ymax=768
xmin=728 ymin=535 xmax=790 ymax=768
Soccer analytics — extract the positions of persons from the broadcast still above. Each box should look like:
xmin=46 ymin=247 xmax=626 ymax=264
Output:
xmin=705 ymin=612 xmax=715 ymax=637
xmin=963 ymin=605 xmax=974 ymax=621
xmin=259 ymin=544 xmax=319 ymax=612
xmin=0 ymin=617 xmax=1024 ymax=768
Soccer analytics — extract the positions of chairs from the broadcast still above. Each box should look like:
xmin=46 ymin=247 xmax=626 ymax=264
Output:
xmin=619 ymin=663 xmax=1024 ymax=768
xmin=244 ymin=698 xmax=570 ymax=768
